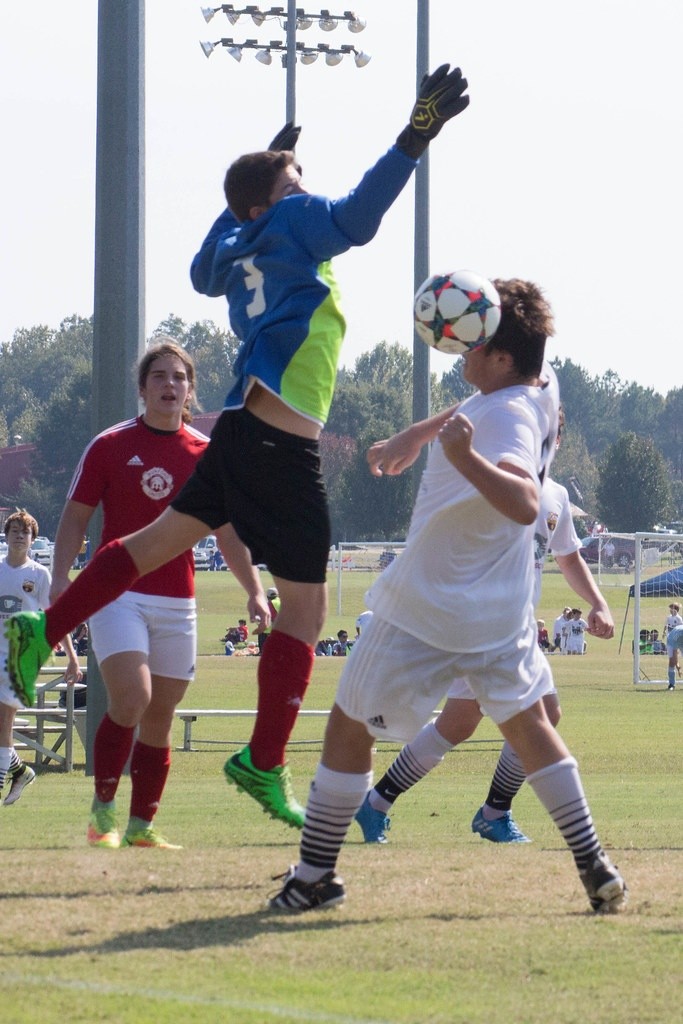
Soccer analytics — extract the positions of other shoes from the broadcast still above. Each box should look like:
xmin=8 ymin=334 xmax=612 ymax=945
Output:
xmin=668 ymin=683 xmax=676 ymax=691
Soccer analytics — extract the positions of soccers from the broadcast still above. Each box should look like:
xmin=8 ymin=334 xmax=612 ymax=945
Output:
xmin=413 ymin=268 xmax=502 ymax=357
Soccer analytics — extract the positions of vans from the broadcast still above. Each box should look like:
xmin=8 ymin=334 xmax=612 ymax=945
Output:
xmin=191 ymin=534 xmax=230 ymax=571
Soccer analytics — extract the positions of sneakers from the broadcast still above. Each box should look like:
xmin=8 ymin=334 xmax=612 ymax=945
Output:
xmin=471 ymin=806 xmax=535 ymax=844
xmin=4 ymin=763 xmax=36 ymax=806
xmin=579 ymin=856 xmax=630 ymax=913
xmin=120 ymin=825 xmax=182 ymax=850
xmin=266 ymin=864 xmax=348 ymax=916
xmin=4 ymin=607 xmax=54 ymax=709
xmin=86 ymin=796 xmax=122 ymax=847
xmin=222 ymin=743 xmax=306 ymax=831
xmin=354 ymin=790 xmax=392 ymax=844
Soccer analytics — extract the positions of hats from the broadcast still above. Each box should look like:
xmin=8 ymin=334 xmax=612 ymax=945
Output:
xmin=267 ymin=587 xmax=279 ymax=598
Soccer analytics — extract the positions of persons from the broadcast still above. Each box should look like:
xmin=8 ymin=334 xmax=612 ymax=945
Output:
xmin=312 ymin=610 xmax=374 ymax=657
xmin=554 ymin=606 xmax=591 ymax=655
xmin=220 ymin=586 xmax=282 ymax=657
xmin=353 ymin=409 xmax=615 ymax=844
xmin=45 ymin=343 xmax=271 ymax=851
xmin=9 ymin=62 xmax=470 ymax=823
xmin=662 ymin=602 xmax=683 ymax=692
xmin=639 ymin=629 xmax=665 ymax=655
xmin=208 ymin=545 xmax=224 ymax=572
xmin=0 ymin=510 xmax=82 ymax=804
xmin=259 ymin=274 xmax=626 ymax=911
xmin=78 ymin=533 xmax=92 ymax=571
xmin=603 ymin=539 xmax=615 ymax=570
xmin=536 ymin=619 xmax=552 ymax=652
xmin=33 ymin=552 xmax=41 ymax=564
xmin=54 ymin=620 xmax=89 ymax=656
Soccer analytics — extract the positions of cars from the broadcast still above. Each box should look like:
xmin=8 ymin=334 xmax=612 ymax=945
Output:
xmin=579 ymin=535 xmax=636 ymax=569
xmin=0 ymin=533 xmax=54 ymax=565
xmin=643 ymin=523 xmax=683 ymax=560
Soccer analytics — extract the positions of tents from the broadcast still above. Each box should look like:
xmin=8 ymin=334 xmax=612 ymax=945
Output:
xmin=618 ymin=566 xmax=683 ymax=657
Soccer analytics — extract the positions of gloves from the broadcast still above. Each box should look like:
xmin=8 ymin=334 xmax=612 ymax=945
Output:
xmin=270 ymin=122 xmax=301 ymax=151
xmin=396 ymin=63 xmax=470 ymax=162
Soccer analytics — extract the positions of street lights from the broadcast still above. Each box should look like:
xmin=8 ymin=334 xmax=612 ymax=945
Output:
xmin=199 ymin=0 xmax=372 ymax=154
